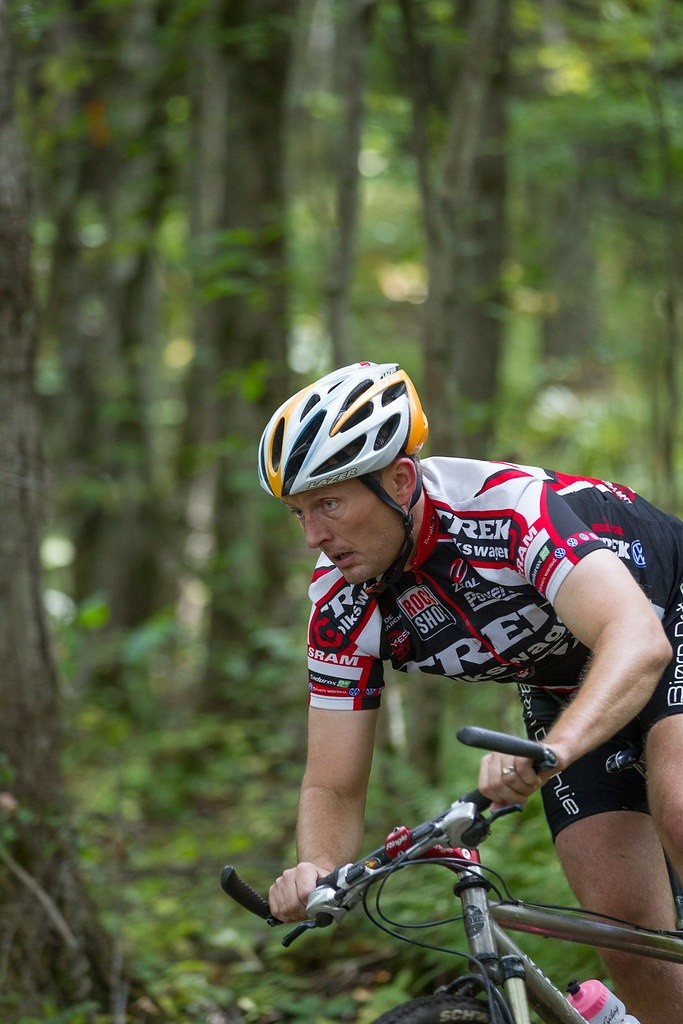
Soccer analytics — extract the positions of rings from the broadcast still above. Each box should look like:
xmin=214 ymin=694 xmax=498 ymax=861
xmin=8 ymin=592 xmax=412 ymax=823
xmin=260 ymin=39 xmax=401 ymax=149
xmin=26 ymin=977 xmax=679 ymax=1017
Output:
xmin=501 ymin=766 xmax=516 ymax=775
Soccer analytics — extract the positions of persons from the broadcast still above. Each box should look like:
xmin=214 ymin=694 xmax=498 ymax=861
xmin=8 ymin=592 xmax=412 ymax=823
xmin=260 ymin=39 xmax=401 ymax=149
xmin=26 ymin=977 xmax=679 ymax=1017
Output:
xmin=258 ymin=361 xmax=683 ymax=1024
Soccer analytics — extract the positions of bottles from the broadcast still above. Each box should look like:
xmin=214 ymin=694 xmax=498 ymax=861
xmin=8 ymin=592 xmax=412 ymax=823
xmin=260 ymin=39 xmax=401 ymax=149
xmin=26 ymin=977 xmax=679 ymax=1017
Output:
xmin=565 ymin=979 xmax=641 ymax=1024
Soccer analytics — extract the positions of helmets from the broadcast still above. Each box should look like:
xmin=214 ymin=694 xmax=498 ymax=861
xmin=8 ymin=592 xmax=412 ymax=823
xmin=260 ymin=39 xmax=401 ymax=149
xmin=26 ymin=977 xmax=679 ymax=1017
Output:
xmin=258 ymin=360 xmax=428 ymax=498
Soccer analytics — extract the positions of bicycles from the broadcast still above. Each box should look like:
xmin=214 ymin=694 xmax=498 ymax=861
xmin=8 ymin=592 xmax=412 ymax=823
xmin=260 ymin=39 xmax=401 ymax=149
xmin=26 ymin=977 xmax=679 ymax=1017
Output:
xmin=219 ymin=724 xmax=683 ymax=1024
